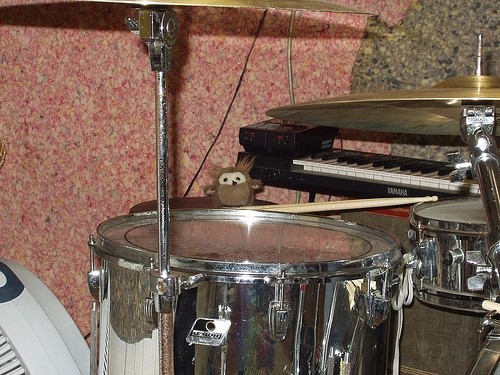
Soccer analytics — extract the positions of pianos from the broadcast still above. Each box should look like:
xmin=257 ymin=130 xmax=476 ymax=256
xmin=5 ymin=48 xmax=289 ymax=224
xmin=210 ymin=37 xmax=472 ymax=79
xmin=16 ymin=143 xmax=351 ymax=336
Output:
xmin=241 ymin=140 xmax=484 ymax=208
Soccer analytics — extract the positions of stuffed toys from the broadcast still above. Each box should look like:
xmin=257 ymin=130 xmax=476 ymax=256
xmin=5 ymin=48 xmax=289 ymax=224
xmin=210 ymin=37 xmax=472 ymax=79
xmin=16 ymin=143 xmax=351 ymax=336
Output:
xmin=204 ymin=153 xmax=263 ymax=209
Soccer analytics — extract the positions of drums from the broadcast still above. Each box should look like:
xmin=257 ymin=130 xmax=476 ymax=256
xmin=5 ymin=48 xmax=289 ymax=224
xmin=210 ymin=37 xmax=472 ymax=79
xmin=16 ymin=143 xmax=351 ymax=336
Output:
xmin=403 ymin=187 xmax=500 ymax=318
xmin=76 ymin=206 xmax=397 ymax=375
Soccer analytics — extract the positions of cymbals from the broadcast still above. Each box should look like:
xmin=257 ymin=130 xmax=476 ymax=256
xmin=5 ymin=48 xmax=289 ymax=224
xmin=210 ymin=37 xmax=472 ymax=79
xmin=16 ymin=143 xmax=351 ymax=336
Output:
xmin=262 ymin=67 xmax=500 ymax=122
xmin=8 ymin=0 xmax=378 ymax=22
